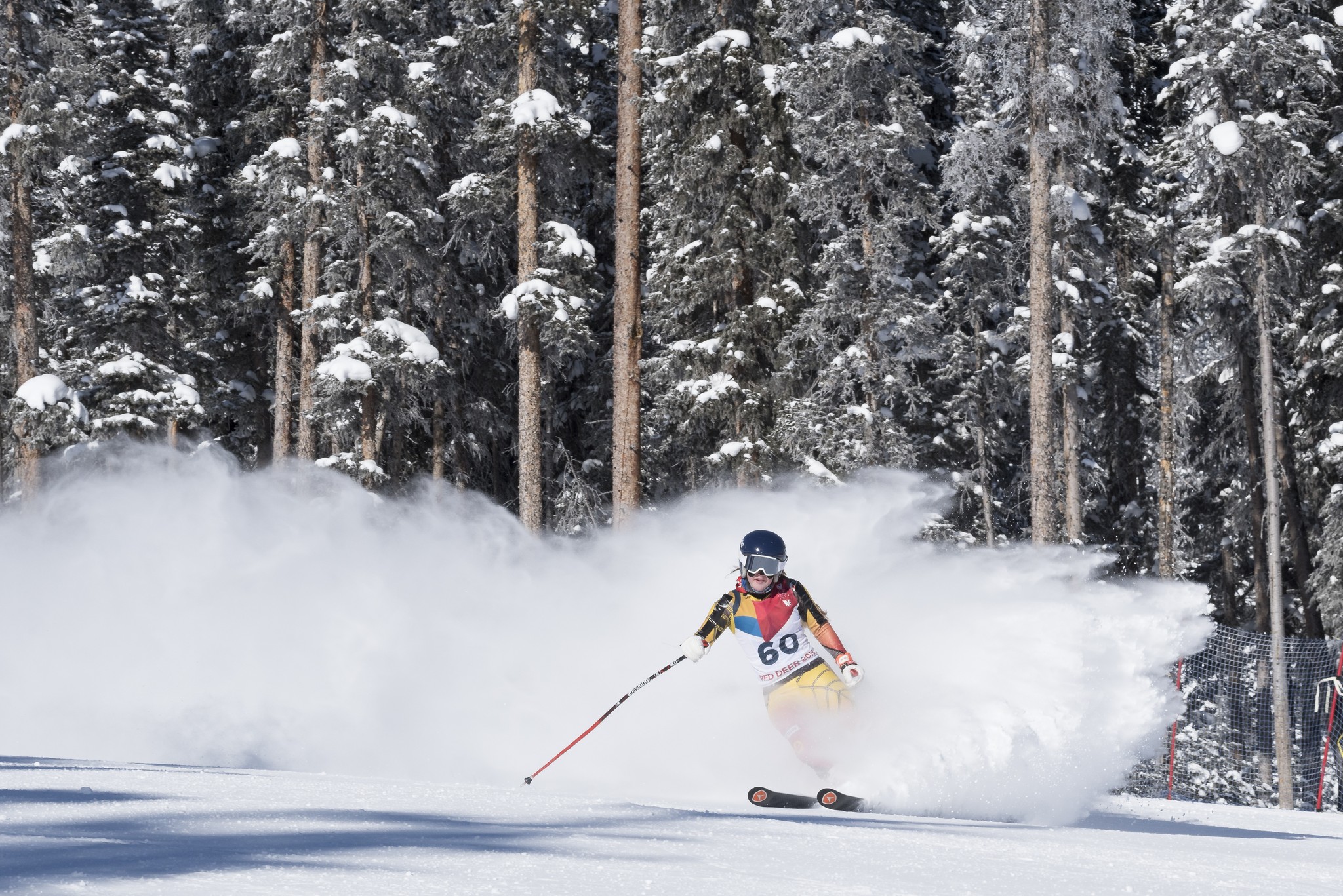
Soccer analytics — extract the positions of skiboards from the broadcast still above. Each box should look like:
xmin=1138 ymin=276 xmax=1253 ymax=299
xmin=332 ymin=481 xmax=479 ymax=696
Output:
xmin=747 ymin=786 xmax=865 ymax=813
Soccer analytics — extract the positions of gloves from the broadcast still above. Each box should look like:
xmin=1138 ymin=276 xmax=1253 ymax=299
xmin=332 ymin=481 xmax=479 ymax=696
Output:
xmin=680 ymin=635 xmax=710 ymax=661
xmin=835 ymin=652 xmax=864 ymax=689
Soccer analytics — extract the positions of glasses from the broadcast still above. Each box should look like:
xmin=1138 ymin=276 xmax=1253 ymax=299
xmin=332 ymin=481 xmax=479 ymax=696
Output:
xmin=745 ymin=554 xmax=781 ymax=576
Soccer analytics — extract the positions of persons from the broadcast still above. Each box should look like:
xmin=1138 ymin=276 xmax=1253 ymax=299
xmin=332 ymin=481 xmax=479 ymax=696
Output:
xmin=680 ymin=529 xmax=866 ymax=778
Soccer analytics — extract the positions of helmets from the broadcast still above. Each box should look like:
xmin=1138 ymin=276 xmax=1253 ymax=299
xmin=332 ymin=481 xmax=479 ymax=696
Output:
xmin=739 ymin=529 xmax=786 ymax=582
